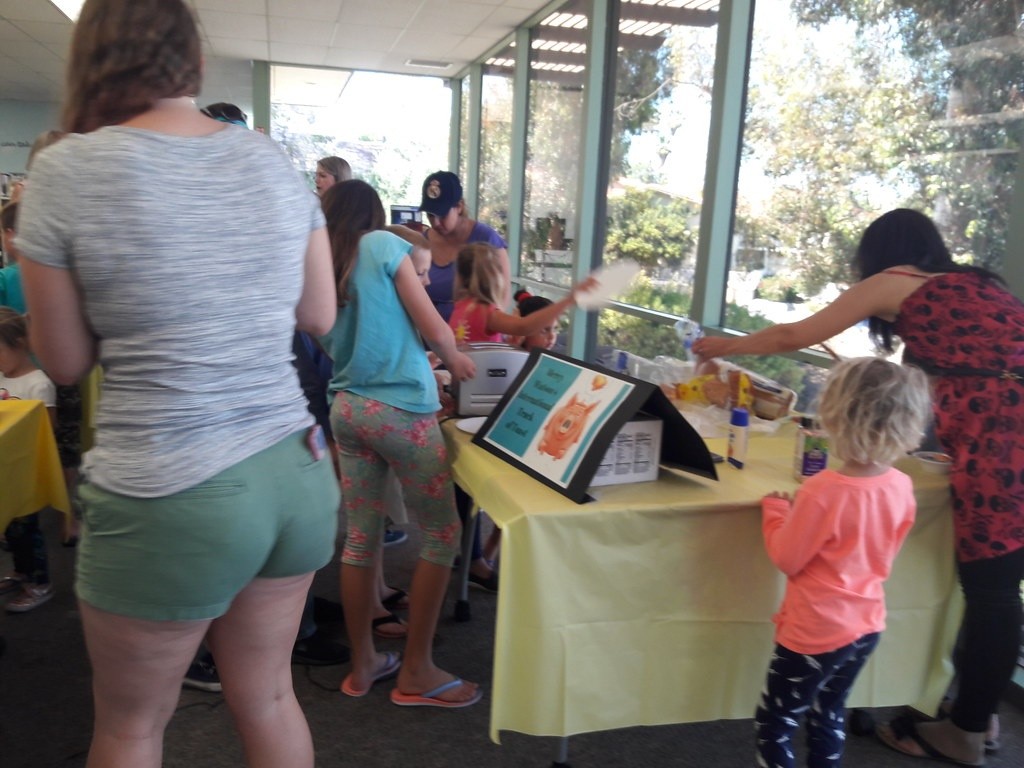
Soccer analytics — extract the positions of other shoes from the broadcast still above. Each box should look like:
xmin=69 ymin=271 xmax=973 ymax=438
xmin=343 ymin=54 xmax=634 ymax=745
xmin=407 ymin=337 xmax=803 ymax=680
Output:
xmin=183 ymin=660 xmax=222 ymax=691
xmin=0 ymin=575 xmax=52 ymax=610
xmin=468 ymin=568 xmax=498 ymax=594
xmin=292 ymin=632 xmax=343 ymax=665
xmin=383 ymin=530 xmax=407 ymax=546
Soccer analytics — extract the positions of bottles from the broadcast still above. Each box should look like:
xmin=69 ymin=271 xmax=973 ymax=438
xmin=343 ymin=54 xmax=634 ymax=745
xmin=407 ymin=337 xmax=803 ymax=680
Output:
xmin=727 ymin=406 xmax=749 ymax=469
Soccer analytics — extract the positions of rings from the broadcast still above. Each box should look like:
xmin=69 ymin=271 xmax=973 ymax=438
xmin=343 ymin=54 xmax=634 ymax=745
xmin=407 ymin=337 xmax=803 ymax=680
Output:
xmin=698 ymin=348 xmax=703 ymax=356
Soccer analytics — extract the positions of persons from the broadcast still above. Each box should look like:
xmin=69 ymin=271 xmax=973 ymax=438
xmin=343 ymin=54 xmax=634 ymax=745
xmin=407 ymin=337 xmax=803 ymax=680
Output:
xmin=22 ymin=0 xmax=336 ymax=768
xmin=693 ymin=207 xmax=1024 ymax=768
xmin=0 ymin=203 xmax=84 ymax=546
xmin=179 ymin=104 xmax=245 ymax=690
xmin=0 ymin=304 xmax=52 ymax=614
xmin=294 ymin=157 xmax=485 ymax=708
xmin=415 ymin=172 xmax=600 ymax=595
xmin=755 ymin=356 xmax=932 ymax=768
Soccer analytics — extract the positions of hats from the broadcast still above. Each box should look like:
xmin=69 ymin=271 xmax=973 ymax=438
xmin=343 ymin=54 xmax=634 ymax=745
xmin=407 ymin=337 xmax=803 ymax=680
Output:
xmin=418 ymin=171 xmax=462 ymax=218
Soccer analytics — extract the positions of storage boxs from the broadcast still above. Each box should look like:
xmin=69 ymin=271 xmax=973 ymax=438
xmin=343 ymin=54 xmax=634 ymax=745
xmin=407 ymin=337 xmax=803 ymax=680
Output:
xmin=590 ymin=418 xmax=664 ymax=485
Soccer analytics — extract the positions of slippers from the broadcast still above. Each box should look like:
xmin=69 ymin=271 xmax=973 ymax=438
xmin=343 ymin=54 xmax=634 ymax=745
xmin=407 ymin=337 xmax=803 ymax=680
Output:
xmin=382 ymin=586 xmax=409 ymax=609
xmin=984 ymin=738 xmax=1001 ymax=752
xmin=341 ymin=651 xmax=401 ymax=696
xmin=874 ymin=718 xmax=986 ymax=767
xmin=372 ymin=614 xmax=407 ymax=638
xmin=391 ymin=677 xmax=483 ymax=708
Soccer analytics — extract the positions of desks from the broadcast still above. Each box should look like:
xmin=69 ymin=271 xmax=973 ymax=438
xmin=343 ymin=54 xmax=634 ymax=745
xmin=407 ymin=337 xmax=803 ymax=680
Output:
xmin=437 ymin=416 xmax=968 ymax=768
xmin=0 ymin=400 xmax=71 ymax=538
